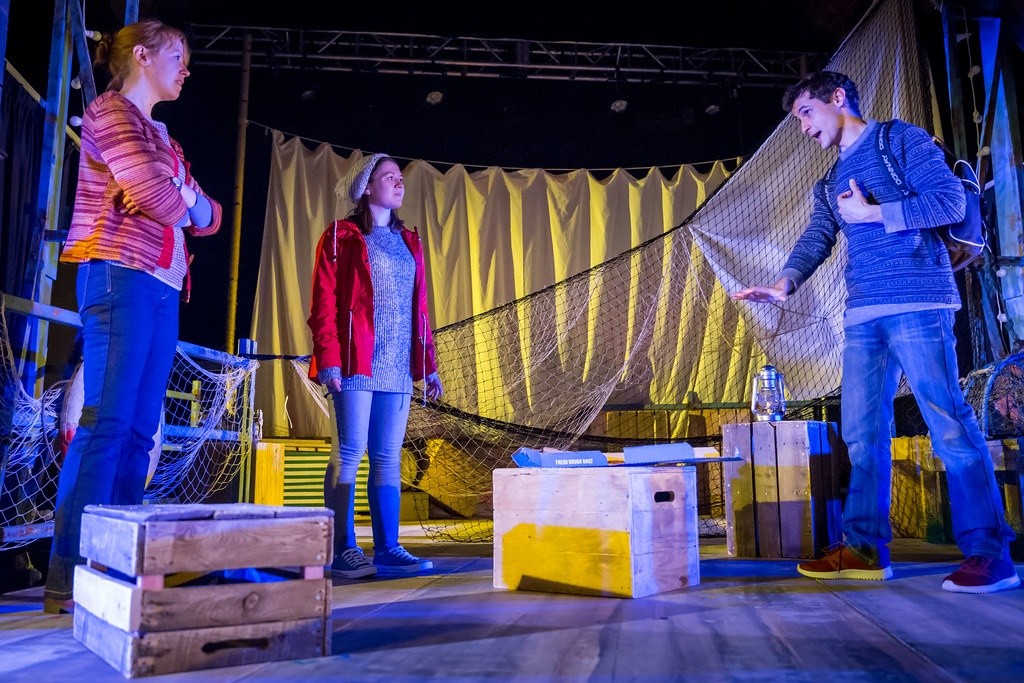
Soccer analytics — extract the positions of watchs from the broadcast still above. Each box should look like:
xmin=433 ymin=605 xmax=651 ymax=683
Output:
xmin=171 ymin=177 xmax=182 ymax=192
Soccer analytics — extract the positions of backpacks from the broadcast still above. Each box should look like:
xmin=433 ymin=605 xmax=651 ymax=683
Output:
xmin=823 ymin=121 xmax=988 ymax=272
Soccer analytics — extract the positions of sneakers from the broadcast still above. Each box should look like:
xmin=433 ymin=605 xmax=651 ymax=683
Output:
xmin=331 ymin=547 xmax=377 ymax=578
xmin=372 ymin=544 xmax=433 ymax=573
xmin=942 ymin=556 xmax=1020 ymax=594
xmin=797 ymin=542 xmax=893 ymax=580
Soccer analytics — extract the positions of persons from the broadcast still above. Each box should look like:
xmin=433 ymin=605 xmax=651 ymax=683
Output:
xmin=41 ymin=21 xmax=225 ymax=616
xmin=731 ymin=70 xmax=1020 ymax=594
xmin=306 ymin=154 xmax=443 ymax=580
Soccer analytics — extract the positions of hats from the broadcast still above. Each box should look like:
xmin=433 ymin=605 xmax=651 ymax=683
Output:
xmin=335 ymin=154 xmax=392 ymax=204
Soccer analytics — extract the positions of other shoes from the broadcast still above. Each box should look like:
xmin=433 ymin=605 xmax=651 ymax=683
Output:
xmin=44 ymin=598 xmax=74 ymax=614
